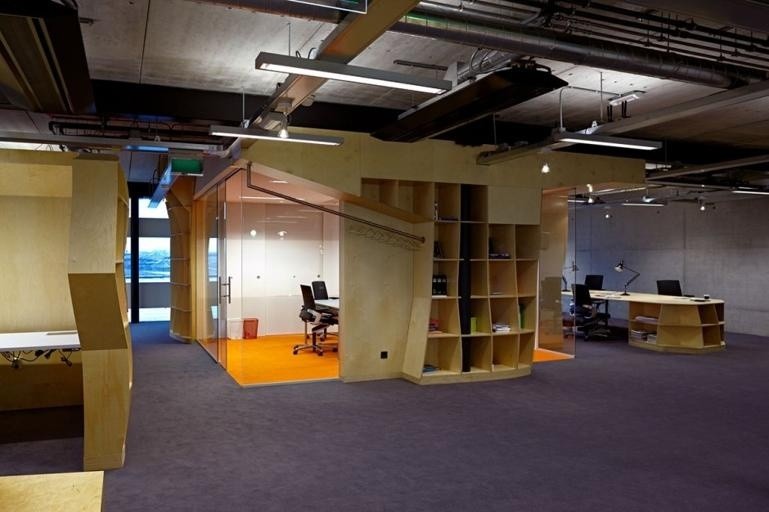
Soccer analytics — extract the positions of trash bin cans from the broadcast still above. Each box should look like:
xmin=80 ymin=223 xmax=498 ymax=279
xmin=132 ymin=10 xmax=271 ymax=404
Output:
xmin=227 ymin=318 xmax=258 ymax=340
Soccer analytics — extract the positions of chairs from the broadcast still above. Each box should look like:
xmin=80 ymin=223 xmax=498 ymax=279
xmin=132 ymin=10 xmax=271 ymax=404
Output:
xmin=293 ymin=281 xmax=339 ymax=355
xmin=584 ymin=275 xmax=611 ymax=337
xmin=569 ymin=283 xmax=608 ymax=338
xmin=657 ymin=280 xmax=695 ymax=297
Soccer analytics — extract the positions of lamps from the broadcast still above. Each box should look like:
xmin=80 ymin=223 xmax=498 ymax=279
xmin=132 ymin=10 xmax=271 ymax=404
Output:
xmin=255 ymin=52 xmax=451 ymax=95
xmin=614 ymin=259 xmax=640 ymax=296
xmin=209 ymin=125 xmax=345 ymax=146
xmin=555 ymin=132 xmax=662 ymax=151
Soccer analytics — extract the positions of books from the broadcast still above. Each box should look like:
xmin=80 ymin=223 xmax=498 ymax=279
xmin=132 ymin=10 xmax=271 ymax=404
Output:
xmin=428 ymin=273 xmax=448 ymax=332
xmin=491 ymin=321 xmax=513 ymax=333
xmin=421 ymin=364 xmax=441 ymax=372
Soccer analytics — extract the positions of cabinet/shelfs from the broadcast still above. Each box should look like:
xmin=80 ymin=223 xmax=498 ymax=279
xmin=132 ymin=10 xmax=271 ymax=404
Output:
xmin=164 ymin=175 xmax=197 ymax=343
xmin=359 ymin=178 xmax=539 ymax=385
xmin=66 ymin=152 xmax=132 ymax=470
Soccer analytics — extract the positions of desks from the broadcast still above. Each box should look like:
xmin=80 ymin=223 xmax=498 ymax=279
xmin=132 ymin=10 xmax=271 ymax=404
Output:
xmin=561 ymin=289 xmax=728 ymax=355
xmin=0 ymin=329 xmax=85 ymax=412
